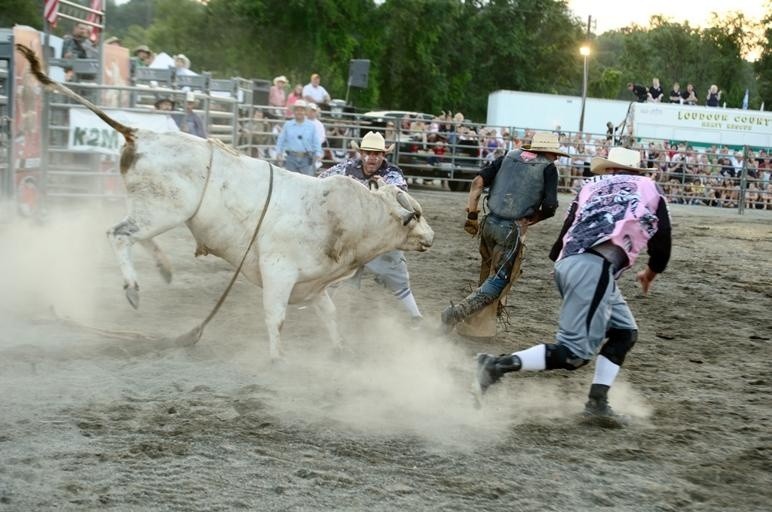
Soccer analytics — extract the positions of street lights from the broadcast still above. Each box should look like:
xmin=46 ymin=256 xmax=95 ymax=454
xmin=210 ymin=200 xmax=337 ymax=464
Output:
xmin=578 ymin=44 xmax=591 ymax=130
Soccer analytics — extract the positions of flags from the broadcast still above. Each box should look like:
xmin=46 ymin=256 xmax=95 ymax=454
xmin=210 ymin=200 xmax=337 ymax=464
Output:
xmin=44 ymin=1 xmax=58 ymax=27
xmin=87 ymin=0 xmax=101 ymax=41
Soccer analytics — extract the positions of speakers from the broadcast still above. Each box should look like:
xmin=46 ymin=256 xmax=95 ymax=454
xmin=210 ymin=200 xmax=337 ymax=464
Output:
xmin=348 ymin=58 xmax=371 ymax=90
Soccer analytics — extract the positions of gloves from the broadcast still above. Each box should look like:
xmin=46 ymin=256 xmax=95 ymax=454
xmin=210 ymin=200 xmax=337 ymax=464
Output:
xmin=465 ymin=206 xmax=481 ymax=235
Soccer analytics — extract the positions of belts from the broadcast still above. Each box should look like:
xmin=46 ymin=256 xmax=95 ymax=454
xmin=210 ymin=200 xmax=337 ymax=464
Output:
xmin=287 ymin=150 xmax=308 ymax=158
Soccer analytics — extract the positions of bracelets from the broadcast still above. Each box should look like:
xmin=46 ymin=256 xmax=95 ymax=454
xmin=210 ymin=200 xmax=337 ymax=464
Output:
xmin=467 ymin=212 xmax=478 ymax=220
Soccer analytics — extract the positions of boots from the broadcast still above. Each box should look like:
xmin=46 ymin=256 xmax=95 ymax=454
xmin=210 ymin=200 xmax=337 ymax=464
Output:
xmin=442 ymin=287 xmax=494 ymax=334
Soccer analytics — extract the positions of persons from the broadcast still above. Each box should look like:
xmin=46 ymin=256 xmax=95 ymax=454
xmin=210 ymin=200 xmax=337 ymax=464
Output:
xmin=317 ymin=130 xmax=426 ymax=333
xmin=438 ymin=131 xmax=571 ymax=342
xmin=476 ymin=147 xmax=672 ymax=422
xmin=47 ymin=23 xmax=772 ymax=211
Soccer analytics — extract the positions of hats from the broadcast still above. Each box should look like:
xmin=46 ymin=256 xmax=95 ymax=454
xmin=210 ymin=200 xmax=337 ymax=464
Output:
xmin=186 ymin=92 xmax=200 ymax=106
xmin=591 ymin=147 xmax=657 ymax=174
xmin=351 ymin=131 xmax=396 ymax=153
xmin=155 ymin=97 xmax=175 ymax=111
xmin=521 ymin=131 xmax=569 ymax=158
xmin=172 ymin=54 xmax=191 ymax=68
xmin=133 ymin=45 xmax=153 ymax=56
xmin=274 ymin=73 xmax=321 ymax=111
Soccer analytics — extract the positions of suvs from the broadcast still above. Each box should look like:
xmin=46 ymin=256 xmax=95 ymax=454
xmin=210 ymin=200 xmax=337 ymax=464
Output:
xmin=343 ymin=110 xmax=489 ymax=192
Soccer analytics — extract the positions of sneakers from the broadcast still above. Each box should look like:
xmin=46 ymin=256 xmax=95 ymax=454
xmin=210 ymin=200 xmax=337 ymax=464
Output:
xmin=476 ymin=351 xmax=499 ymax=392
xmin=584 ymin=402 xmax=631 ymax=428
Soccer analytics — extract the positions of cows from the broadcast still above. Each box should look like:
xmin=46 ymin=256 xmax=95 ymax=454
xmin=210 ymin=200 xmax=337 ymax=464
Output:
xmin=17 ymin=42 xmax=436 ymax=376
